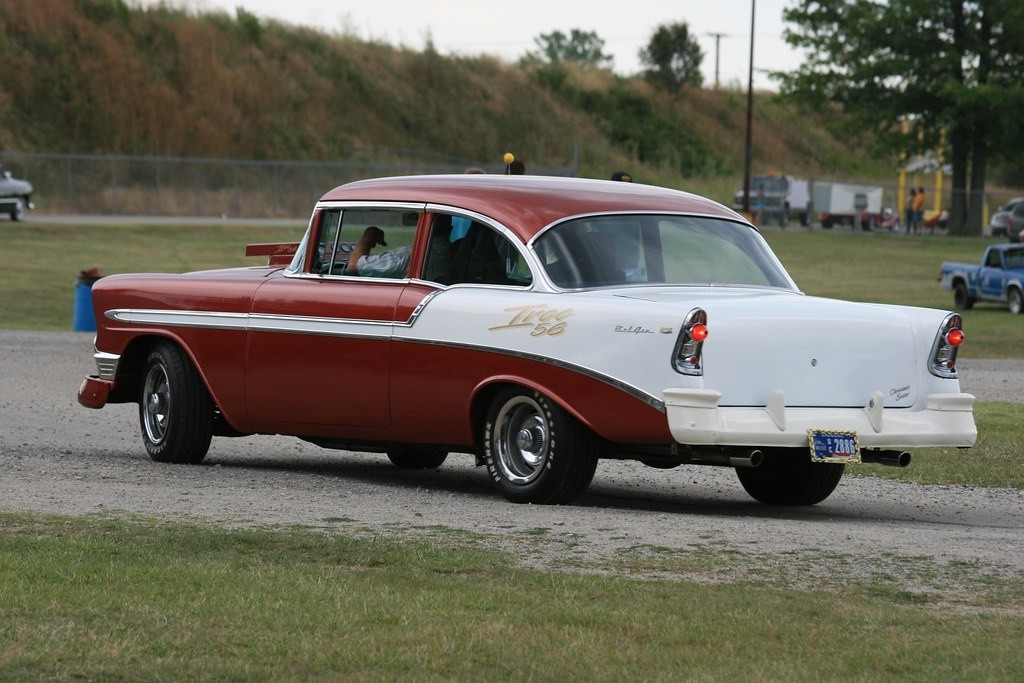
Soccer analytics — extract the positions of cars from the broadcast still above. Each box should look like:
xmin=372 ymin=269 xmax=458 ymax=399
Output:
xmin=79 ymin=171 xmax=983 ymax=505
xmin=1006 ymin=199 xmax=1024 ymax=240
xmin=990 ymin=197 xmax=1024 ymax=238
xmin=0 ymin=169 xmax=37 ymax=222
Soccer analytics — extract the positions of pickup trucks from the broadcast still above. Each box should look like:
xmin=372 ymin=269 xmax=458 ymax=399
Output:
xmin=936 ymin=241 xmax=1024 ymax=315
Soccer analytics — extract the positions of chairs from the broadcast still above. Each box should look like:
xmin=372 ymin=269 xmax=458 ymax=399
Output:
xmin=433 ymin=237 xmax=530 ymax=286
xmin=547 ymin=231 xmax=626 ymax=288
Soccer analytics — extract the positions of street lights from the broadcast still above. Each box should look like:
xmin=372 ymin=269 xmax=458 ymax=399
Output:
xmin=705 ymin=29 xmax=728 ymax=86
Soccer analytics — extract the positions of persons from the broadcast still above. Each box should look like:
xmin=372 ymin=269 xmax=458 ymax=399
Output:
xmin=756 ymin=182 xmax=950 ymax=236
xmin=451 ymin=218 xmax=480 ymax=283
xmin=346 ymin=213 xmax=451 ymax=285
xmin=448 ymin=167 xmax=487 ymax=240
xmin=589 ymin=173 xmax=643 ymax=271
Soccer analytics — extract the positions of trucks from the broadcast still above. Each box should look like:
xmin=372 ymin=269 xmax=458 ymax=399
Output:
xmin=812 ymin=179 xmax=901 ymax=231
xmin=733 ymin=172 xmax=814 ymax=227
